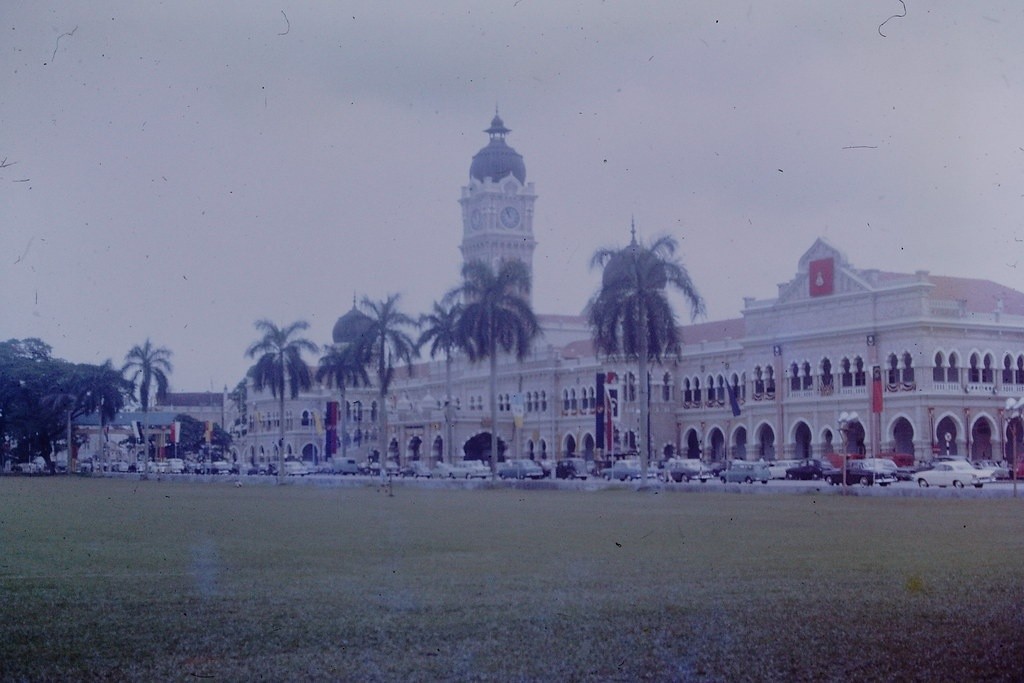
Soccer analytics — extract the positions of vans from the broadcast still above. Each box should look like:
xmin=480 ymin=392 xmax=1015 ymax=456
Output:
xmin=719 ymin=461 xmax=773 ymax=485
xmin=330 ymin=457 xmax=359 ymax=476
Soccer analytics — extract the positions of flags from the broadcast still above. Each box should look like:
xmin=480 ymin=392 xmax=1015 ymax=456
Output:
xmin=595 ymin=373 xmax=613 ymax=451
xmin=205 ymin=420 xmax=213 ymax=442
xmin=725 ymin=378 xmax=740 ymax=416
xmin=132 ymin=421 xmax=143 ymax=438
xmin=170 ymin=422 xmax=180 ymax=443
xmin=104 ymin=427 xmax=110 ymax=442
xmin=326 ymin=401 xmax=338 ymax=458
xmin=872 ymin=366 xmax=883 ymax=413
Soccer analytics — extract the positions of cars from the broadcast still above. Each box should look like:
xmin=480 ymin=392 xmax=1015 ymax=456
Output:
xmin=765 ymin=460 xmax=802 ymax=480
xmin=448 ymin=460 xmax=491 ymax=481
xmin=429 ymin=462 xmax=453 ymax=480
xmin=399 ymin=461 xmax=433 ymax=480
xmin=586 ymin=460 xmax=597 ymax=474
xmin=1016 ymin=458 xmax=1024 ymax=480
xmin=657 ymin=458 xmax=716 ymax=484
xmin=785 ymin=458 xmax=832 ymax=481
xmin=301 ymin=460 xmax=319 ymax=475
xmin=273 ymin=462 xmax=309 ymax=477
xmin=246 ymin=463 xmax=271 ymax=477
xmin=820 ymin=459 xmax=899 ymax=488
xmin=497 ymin=458 xmax=543 ymax=480
xmin=912 ymin=460 xmax=996 ymax=490
xmin=600 ymin=459 xmax=657 ymax=482
xmin=385 ymin=460 xmax=399 ymax=477
xmin=81 ymin=456 xmax=229 ymax=476
xmin=974 ymin=459 xmax=1014 ymax=481
xmin=542 ymin=458 xmax=588 ymax=481
